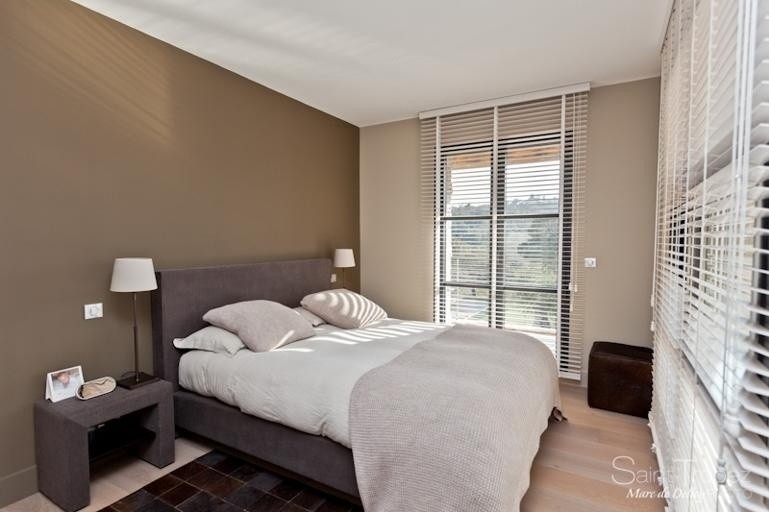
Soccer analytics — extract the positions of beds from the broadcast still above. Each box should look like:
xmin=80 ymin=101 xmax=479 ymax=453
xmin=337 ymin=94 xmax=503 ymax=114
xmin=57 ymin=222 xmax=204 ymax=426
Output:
xmin=153 ymin=258 xmax=568 ymax=511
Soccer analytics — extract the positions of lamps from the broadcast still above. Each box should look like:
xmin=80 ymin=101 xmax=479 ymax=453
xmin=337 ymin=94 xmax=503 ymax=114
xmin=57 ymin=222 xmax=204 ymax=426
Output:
xmin=333 ymin=249 xmax=356 ymax=288
xmin=110 ymin=257 xmax=161 ymax=390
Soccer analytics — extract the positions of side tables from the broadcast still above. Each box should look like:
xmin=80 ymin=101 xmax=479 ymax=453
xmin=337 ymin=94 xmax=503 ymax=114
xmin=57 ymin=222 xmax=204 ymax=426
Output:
xmin=34 ymin=375 xmax=175 ymax=512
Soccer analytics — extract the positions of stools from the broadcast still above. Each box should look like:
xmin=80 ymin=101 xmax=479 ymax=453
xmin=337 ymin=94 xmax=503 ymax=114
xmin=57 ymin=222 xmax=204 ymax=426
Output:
xmin=587 ymin=341 xmax=654 ymax=419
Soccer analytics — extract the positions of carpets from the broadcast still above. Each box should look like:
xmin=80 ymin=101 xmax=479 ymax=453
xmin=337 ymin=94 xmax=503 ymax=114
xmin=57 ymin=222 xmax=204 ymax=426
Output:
xmin=97 ymin=447 xmax=364 ymax=512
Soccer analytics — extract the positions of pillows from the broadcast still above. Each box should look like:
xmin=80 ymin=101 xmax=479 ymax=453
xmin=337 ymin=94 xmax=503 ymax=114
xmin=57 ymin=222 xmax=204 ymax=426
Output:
xmin=173 ymin=326 xmax=246 ymax=356
xmin=292 ymin=307 xmax=326 ymax=326
xmin=202 ymin=300 xmax=317 ymax=353
xmin=300 ymin=288 xmax=388 ymax=329
